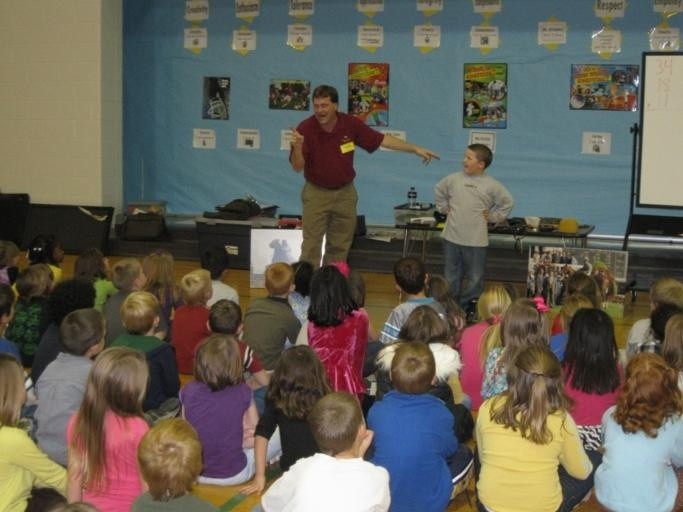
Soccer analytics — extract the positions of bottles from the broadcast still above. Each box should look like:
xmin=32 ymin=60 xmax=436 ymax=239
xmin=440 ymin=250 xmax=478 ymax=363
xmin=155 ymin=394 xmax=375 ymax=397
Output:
xmin=407 ymin=186 xmax=417 ymax=211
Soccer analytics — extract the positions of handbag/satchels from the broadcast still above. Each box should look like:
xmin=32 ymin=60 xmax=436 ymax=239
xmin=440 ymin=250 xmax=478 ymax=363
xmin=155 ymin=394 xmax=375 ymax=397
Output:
xmin=119 ymin=211 xmax=167 ymax=242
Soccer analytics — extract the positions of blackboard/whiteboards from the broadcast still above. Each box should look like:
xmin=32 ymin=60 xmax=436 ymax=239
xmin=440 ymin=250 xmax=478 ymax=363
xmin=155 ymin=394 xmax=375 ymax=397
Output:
xmin=636 ymin=51 xmax=683 ymax=208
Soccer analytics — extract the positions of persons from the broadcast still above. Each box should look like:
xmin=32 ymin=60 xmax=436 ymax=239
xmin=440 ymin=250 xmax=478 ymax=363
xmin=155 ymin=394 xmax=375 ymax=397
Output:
xmin=0 ymin=234 xmax=682 ymax=512
xmin=282 ymin=82 xmax=440 ymax=272
xmin=435 ymin=142 xmax=515 ymax=306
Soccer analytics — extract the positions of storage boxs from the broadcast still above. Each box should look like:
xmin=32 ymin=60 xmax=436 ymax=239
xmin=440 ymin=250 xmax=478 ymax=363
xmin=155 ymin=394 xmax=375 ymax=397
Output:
xmin=193 ymin=214 xmax=298 ymax=271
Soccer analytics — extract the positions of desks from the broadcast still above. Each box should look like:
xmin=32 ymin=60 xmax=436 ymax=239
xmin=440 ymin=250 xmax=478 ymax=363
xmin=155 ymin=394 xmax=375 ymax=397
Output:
xmin=393 ymin=214 xmax=595 ymax=304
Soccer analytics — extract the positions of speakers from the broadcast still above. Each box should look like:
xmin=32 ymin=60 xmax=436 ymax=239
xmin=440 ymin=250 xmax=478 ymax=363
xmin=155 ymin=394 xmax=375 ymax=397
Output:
xmin=0 ymin=193 xmax=28 ymax=250
xmin=20 ymin=203 xmax=115 ymax=255
